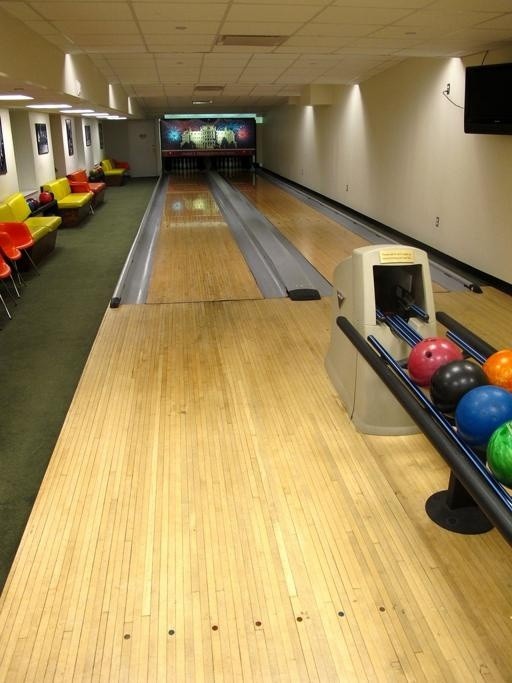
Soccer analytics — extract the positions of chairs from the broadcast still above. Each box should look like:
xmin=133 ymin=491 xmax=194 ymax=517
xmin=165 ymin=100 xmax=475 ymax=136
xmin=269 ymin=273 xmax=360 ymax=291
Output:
xmin=0 ymin=159 xmax=129 ymax=321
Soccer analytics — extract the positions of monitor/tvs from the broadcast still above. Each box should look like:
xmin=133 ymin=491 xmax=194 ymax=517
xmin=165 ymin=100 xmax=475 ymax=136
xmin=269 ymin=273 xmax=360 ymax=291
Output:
xmin=463 ymin=62 xmax=512 ymax=136
